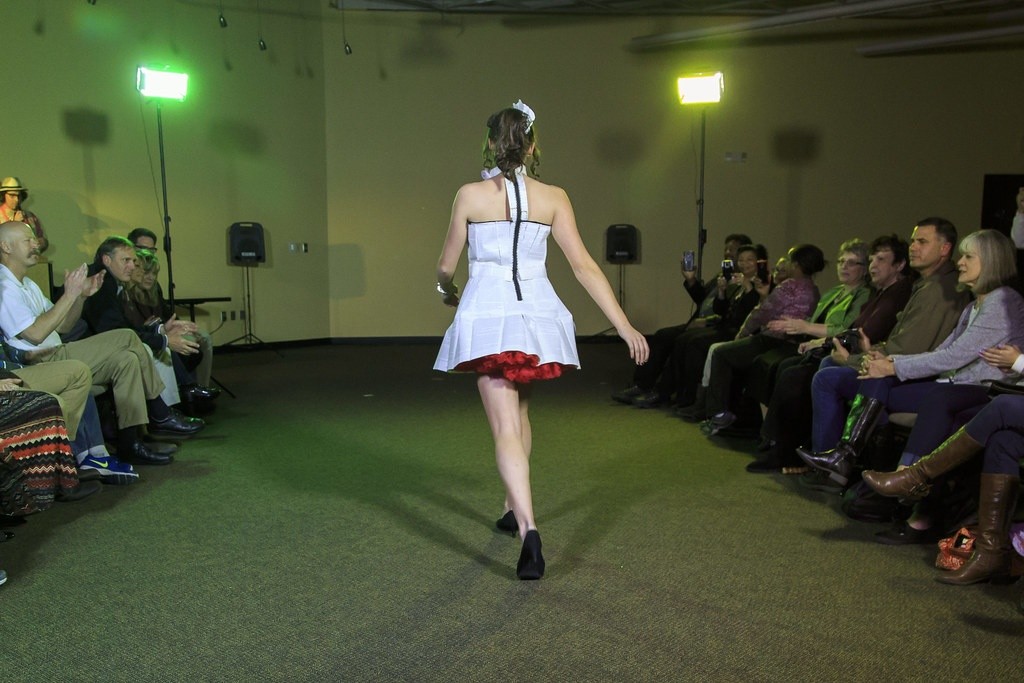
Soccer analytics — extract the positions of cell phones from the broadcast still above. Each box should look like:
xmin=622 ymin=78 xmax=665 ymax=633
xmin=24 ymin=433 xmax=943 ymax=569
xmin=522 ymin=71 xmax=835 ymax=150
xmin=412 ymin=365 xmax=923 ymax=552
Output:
xmin=684 ymin=251 xmax=694 ymax=271
xmin=722 ymin=260 xmax=734 ymax=282
xmin=758 ymin=260 xmax=768 ymax=284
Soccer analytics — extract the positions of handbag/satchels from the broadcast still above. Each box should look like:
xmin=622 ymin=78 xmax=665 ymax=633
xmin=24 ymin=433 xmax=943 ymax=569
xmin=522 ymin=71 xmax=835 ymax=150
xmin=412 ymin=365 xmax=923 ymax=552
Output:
xmin=935 ymin=526 xmax=979 ymax=571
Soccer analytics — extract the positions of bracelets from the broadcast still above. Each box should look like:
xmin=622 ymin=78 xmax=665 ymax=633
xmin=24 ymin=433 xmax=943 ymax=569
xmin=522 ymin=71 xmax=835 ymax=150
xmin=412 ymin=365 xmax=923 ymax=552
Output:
xmin=437 ymin=283 xmax=458 ymax=294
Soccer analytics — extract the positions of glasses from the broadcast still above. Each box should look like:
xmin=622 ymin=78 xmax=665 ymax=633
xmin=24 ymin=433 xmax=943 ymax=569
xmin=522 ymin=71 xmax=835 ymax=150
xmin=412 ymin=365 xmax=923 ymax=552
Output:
xmin=838 ymin=259 xmax=865 ymax=267
xmin=135 ymin=245 xmax=157 ymax=253
xmin=5 ymin=192 xmax=20 ymax=198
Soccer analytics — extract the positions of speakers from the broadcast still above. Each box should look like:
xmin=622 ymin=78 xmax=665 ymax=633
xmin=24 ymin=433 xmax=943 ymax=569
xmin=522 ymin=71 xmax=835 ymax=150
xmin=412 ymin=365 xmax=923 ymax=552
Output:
xmin=605 ymin=224 xmax=638 ymax=264
xmin=230 ymin=221 xmax=265 ymax=267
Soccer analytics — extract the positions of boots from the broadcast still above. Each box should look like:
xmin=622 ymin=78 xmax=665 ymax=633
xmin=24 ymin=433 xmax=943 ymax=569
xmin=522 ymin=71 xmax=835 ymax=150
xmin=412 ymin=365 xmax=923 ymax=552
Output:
xmin=862 ymin=424 xmax=986 ymax=505
xmin=932 ymin=473 xmax=1024 ymax=585
xmin=795 ymin=392 xmax=888 ymax=486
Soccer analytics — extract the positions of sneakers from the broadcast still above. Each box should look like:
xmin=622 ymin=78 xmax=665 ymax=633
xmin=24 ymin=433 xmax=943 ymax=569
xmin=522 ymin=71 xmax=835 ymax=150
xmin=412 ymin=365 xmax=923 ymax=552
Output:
xmin=77 ymin=454 xmax=139 ymax=485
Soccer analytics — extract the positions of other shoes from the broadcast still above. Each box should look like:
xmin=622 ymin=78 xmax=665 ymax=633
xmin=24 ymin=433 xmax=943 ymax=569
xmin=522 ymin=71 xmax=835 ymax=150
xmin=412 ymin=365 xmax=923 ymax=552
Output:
xmin=874 ymin=520 xmax=943 ymax=543
xmin=0 ymin=481 xmax=102 ymax=587
xmin=797 ymin=467 xmax=843 ymax=493
xmin=846 ymin=491 xmax=901 ymax=521
xmin=612 ymin=384 xmax=804 ymax=473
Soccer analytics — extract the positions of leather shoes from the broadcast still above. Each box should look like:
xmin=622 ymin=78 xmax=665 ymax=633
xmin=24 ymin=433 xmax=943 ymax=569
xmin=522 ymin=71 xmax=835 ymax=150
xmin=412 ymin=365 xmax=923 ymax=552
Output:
xmin=147 ymin=409 xmax=204 ymax=434
xmin=117 ymin=442 xmax=171 ymax=465
xmin=76 ymin=469 xmax=100 ymax=483
xmin=177 ymin=382 xmax=221 ymax=405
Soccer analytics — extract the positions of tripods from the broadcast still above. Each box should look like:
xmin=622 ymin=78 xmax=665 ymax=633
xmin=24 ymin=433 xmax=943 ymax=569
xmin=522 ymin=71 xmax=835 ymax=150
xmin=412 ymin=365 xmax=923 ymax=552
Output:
xmin=212 ymin=267 xmax=286 ymax=359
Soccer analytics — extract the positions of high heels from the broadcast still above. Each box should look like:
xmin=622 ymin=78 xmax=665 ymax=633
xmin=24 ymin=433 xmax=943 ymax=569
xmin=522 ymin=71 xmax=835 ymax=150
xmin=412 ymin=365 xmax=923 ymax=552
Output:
xmin=516 ymin=530 xmax=545 ymax=580
xmin=496 ymin=510 xmax=518 ymax=538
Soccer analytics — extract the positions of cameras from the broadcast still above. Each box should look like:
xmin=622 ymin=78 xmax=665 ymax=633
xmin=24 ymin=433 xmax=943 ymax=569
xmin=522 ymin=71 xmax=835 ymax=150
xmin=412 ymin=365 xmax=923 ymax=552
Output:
xmin=822 ymin=328 xmax=859 ymax=355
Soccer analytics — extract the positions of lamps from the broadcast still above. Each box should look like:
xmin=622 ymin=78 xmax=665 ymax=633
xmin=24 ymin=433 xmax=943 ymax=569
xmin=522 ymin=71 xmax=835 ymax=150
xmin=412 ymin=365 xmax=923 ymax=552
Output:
xmin=677 ymin=66 xmax=725 ymax=285
xmin=135 ymin=65 xmax=189 ymax=313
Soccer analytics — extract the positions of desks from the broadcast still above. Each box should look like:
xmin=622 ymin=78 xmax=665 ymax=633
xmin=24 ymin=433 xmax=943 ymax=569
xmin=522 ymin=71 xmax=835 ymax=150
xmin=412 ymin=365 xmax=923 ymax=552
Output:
xmin=164 ymin=297 xmax=232 ymax=322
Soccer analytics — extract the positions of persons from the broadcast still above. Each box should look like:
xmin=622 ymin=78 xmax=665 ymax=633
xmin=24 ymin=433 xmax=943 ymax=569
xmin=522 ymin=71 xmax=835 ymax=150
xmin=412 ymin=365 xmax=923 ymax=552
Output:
xmin=432 ymin=100 xmax=651 ymax=580
xmin=615 ymin=189 xmax=1024 ymax=587
xmin=0 ymin=176 xmax=220 ymax=584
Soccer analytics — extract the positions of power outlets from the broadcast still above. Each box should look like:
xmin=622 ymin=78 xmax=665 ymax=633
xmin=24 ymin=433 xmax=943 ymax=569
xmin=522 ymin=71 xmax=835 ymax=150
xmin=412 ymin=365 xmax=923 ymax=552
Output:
xmin=222 ymin=312 xmax=226 ymax=321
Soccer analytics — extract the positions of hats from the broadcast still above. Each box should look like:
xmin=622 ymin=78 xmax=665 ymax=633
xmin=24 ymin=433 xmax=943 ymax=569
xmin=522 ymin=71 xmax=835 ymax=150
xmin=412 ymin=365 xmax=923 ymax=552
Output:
xmin=0 ymin=177 xmax=25 ymax=191
